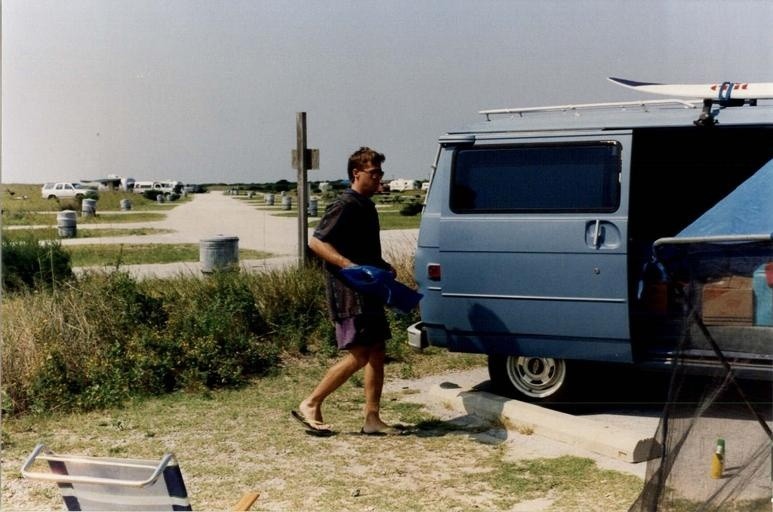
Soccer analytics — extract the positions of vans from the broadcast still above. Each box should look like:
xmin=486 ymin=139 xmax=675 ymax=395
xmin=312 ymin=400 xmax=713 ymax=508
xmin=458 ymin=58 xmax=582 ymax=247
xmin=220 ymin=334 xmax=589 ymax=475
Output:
xmin=406 ymin=78 xmax=773 ymax=404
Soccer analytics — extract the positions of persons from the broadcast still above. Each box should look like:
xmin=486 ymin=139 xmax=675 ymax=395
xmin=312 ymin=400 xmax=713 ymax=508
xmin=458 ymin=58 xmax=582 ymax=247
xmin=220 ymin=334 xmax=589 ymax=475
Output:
xmin=299 ymin=146 xmax=409 ymax=434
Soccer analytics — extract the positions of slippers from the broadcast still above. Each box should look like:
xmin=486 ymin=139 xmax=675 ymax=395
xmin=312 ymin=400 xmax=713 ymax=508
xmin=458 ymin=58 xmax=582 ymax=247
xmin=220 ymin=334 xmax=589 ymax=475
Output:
xmin=291 ymin=410 xmax=332 ymax=436
xmin=360 ymin=426 xmax=409 ymax=437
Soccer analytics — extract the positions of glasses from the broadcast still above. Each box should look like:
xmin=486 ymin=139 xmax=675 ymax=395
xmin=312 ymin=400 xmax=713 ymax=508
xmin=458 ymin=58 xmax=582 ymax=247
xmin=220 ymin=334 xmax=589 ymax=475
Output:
xmin=358 ymin=168 xmax=385 ymax=177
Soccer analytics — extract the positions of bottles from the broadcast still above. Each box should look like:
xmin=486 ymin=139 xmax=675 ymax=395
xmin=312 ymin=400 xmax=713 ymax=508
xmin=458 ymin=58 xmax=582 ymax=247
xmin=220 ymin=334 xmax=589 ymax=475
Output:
xmin=710 ymin=438 xmax=724 ymax=481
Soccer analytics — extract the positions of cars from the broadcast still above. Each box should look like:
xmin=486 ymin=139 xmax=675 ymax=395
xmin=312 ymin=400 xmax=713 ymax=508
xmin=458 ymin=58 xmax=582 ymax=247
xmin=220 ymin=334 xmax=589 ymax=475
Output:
xmin=41 ymin=176 xmax=204 ymax=198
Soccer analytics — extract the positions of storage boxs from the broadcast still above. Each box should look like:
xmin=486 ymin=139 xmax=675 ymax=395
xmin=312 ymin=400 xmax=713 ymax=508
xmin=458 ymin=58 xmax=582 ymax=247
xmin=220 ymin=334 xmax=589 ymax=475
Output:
xmin=700 ymin=273 xmax=756 ymax=326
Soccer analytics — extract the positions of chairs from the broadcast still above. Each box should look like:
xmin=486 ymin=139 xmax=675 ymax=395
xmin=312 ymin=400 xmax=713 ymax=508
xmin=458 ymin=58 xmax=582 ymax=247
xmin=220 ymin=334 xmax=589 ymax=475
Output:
xmin=19 ymin=442 xmax=260 ymax=511
